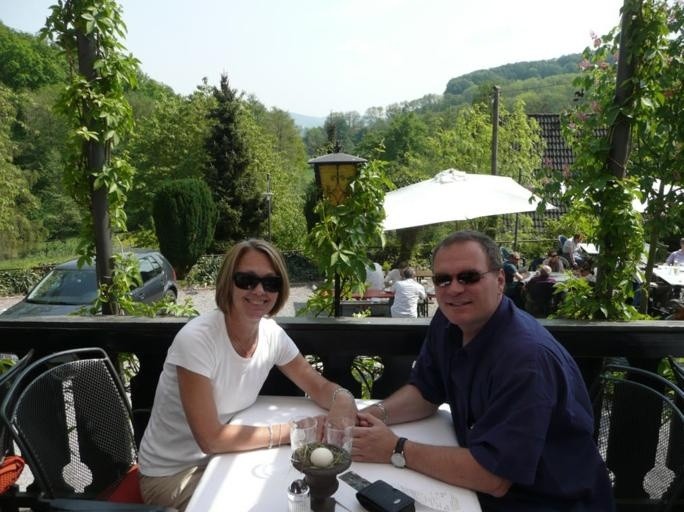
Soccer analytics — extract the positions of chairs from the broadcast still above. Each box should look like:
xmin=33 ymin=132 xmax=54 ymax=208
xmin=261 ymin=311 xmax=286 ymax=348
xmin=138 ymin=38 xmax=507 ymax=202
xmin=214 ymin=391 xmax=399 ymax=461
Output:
xmin=2 ymin=346 xmax=165 ymax=512
xmin=664 ymin=355 xmax=684 ymax=511
xmin=558 ymin=235 xmax=568 ymax=248
xmin=593 ymin=365 xmax=684 ymax=511
xmin=526 ymin=281 xmax=557 ymax=316
xmin=1 ymin=347 xmax=34 ymax=512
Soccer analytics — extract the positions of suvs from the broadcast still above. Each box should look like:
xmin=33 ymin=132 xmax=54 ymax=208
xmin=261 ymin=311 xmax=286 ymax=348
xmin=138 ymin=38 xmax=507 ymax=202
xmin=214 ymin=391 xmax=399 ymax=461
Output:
xmin=1 ymin=251 xmax=178 ymax=318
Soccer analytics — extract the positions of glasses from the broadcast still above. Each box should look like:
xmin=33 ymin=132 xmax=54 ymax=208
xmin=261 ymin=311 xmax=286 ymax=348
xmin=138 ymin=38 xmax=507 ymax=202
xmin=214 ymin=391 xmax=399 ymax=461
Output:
xmin=432 ymin=269 xmax=497 ymax=288
xmin=232 ymin=271 xmax=283 ymax=293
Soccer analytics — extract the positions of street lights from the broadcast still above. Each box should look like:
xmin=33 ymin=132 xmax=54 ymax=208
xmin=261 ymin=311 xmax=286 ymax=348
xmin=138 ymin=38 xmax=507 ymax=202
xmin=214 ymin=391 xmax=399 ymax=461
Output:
xmin=308 ymin=142 xmax=367 ymax=318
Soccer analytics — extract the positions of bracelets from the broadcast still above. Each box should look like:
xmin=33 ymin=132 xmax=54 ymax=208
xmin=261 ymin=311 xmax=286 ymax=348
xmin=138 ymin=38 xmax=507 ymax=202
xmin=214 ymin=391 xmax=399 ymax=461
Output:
xmin=268 ymin=421 xmax=284 ymax=451
xmin=370 ymin=400 xmax=391 ymax=428
xmin=332 ymin=386 xmax=356 ymax=405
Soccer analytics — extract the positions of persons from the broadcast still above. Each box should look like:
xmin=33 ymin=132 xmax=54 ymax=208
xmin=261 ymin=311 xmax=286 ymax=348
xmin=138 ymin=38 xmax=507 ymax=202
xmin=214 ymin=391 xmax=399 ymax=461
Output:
xmin=343 ymin=230 xmax=615 ymax=512
xmin=137 ymin=237 xmax=360 ymax=512
xmin=666 ymin=239 xmax=684 ymax=267
xmin=542 ymin=250 xmax=563 ymax=273
xmin=525 ymin=265 xmax=557 ymax=302
xmin=502 ymin=252 xmax=525 ymax=309
xmin=580 ymin=263 xmax=596 ymax=283
xmin=390 ymin=267 xmax=427 ymax=319
xmin=384 ymin=262 xmax=408 ymax=288
xmin=561 ymin=234 xmax=584 ymax=266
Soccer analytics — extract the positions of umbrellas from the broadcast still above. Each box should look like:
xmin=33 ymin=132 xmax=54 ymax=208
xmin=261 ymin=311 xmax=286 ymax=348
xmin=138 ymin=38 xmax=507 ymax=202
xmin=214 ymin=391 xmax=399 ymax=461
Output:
xmin=380 ymin=167 xmax=560 ymax=231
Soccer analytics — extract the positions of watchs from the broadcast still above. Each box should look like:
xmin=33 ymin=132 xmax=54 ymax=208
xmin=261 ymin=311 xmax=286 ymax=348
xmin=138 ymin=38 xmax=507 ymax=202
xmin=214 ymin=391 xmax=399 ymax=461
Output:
xmin=390 ymin=436 xmax=408 ymax=471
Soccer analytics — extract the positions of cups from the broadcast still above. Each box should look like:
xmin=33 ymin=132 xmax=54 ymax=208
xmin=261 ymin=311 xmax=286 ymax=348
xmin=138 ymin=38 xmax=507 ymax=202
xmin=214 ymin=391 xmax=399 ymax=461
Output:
xmin=325 ymin=417 xmax=355 ymax=455
xmin=289 ymin=415 xmax=318 ymax=451
